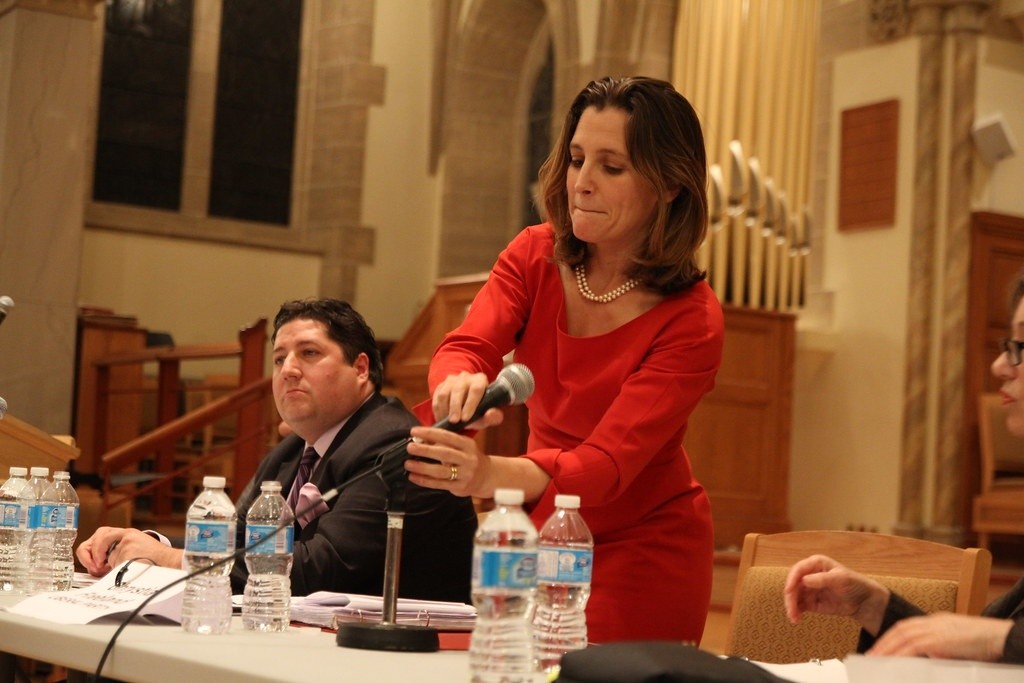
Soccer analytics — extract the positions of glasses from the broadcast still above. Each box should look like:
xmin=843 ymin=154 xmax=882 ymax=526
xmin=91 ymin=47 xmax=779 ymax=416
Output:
xmin=1004 ymin=341 xmax=1024 ymax=365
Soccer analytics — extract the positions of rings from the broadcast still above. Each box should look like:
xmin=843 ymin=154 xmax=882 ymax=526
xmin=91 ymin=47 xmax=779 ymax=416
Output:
xmin=450 ymin=463 xmax=458 ymax=482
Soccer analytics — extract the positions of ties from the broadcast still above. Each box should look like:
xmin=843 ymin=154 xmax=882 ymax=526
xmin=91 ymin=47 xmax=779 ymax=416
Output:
xmin=287 ymin=447 xmax=319 ymax=512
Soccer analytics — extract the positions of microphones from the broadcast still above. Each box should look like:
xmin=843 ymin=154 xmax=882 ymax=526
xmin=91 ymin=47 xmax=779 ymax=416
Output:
xmin=411 ymin=363 xmax=535 ymax=443
xmin=0 ymin=296 xmax=14 ymax=325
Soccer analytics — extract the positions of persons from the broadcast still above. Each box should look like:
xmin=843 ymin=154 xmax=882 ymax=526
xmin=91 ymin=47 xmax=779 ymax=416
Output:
xmin=76 ymin=298 xmax=476 ymax=604
xmin=405 ymin=74 xmax=726 ymax=653
xmin=784 ymin=271 xmax=1024 ymax=682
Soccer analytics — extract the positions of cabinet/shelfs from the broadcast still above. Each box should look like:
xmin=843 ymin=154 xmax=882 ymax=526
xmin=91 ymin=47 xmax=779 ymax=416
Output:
xmin=68 ymin=316 xmax=149 ymax=505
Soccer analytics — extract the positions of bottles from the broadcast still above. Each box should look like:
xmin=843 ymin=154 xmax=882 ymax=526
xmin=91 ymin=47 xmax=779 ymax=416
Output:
xmin=467 ymin=488 xmax=538 ymax=683
xmin=14 ymin=466 xmax=59 ymax=597
xmin=0 ymin=466 xmax=28 ymax=593
xmin=181 ymin=475 xmax=238 ymax=635
xmin=525 ymin=496 xmax=594 ymax=677
xmin=241 ymin=480 xmax=294 ymax=634
xmin=33 ymin=470 xmax=80 ymax=592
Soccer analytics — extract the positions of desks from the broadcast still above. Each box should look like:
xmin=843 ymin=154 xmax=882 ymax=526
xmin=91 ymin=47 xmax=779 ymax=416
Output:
xmin=0 ymin=571 xmax=542 ymax=683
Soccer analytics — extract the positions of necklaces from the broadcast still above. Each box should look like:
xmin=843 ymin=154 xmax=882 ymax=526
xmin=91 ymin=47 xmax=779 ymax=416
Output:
xmin=571 ymin=263 xmax=645 ymax=304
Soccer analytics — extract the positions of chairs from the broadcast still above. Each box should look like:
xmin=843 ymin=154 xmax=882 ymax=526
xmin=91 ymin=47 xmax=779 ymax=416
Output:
xmin=972 ymin=394 xmax=1024 ymax=551
xmin=725 ymin=531 xmax=992 ymax=665
